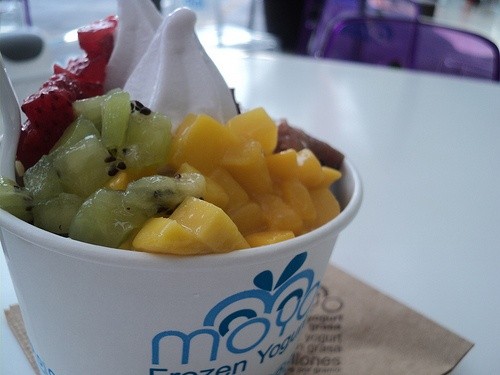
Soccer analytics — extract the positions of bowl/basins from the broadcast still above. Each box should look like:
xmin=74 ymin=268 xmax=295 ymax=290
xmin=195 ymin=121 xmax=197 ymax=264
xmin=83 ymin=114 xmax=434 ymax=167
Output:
xmin=0 ymin=156 xmax=365 ymax=375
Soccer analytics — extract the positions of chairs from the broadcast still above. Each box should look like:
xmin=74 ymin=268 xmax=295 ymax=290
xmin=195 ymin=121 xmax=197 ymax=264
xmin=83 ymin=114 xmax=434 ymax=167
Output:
xmin=309 ymin=11 xmax=499 ymax=84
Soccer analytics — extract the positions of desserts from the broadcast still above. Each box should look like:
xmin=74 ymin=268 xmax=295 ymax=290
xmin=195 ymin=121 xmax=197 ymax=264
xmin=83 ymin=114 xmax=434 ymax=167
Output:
xmin=0 ymin=0 xmax=349 ymax=256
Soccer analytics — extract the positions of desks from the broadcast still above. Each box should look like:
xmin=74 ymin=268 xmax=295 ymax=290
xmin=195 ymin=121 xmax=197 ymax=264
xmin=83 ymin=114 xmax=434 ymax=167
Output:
xmin=0 ymin=25 xmax=499 ymax=375
